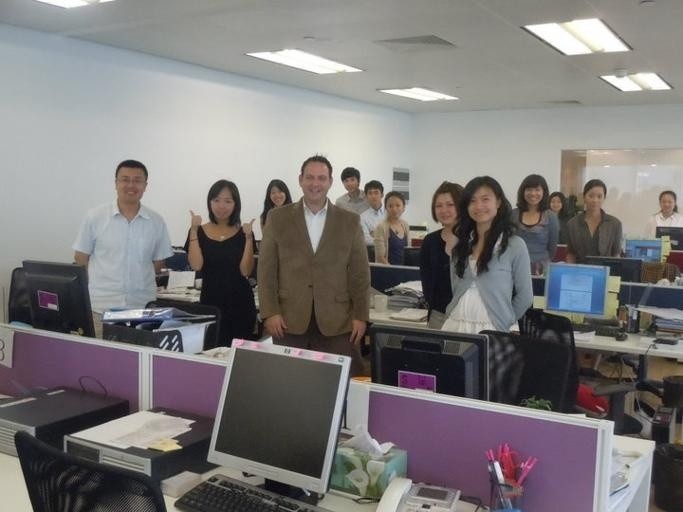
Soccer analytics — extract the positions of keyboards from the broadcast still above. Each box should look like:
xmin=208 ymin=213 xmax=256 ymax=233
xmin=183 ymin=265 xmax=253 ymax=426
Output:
xmin=173 ymin=473 xmax=334 ymax=512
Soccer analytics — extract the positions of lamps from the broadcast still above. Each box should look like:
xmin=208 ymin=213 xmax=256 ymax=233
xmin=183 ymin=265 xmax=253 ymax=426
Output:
xmin=376 ymin=85 xmax=461 ymax=103
xmin=597 ymin=70 xmax=676 ymax=94
xmin=244 ymin=47 xmax=366 ymax=76
xmin=520 ymin=15 xmax=634 ymax=58
xmin=31 ymin=0 xmax=114 ymax=12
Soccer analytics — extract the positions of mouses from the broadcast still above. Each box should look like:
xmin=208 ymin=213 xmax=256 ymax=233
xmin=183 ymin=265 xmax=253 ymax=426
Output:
xmin=615 ymin=332 xmax=628 ymax=341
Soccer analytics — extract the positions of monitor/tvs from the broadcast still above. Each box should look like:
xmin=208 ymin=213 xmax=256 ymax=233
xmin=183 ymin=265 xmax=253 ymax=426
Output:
xmin=624 ymin=238 xmax=663 ymax=263
xmin=22 ymin=259 xmax=96 ymax=338
xmin=206 ymin=337 xmax=354 ymax=505
xmin=584 ymin=255 xmax=644 ymax=283
xmin=656 ymin=226 xmax=683 ymax=250
xmin=401 ymin=245 xmax=422 ymax=267
xmin=543 ymin=262 xmax=610 ymax=316
xmin=369 ymin=322 xmax=491 ymax=402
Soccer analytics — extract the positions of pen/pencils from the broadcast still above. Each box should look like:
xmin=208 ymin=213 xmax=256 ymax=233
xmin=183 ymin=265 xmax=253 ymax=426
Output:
xmin=489 ymin=449 xmax=494 ymax=462
xmin=515 ymin=457 xmax=538 ymax=484
xmin=504 ymin=443 xmax=513 ymax=476
xmin=496 ymin=444 xmax=502 ymax=460
xmin=485 ymin=450 xmax=505 ymax=508
xmin=493 ymin=459 xmax=513 ymax=509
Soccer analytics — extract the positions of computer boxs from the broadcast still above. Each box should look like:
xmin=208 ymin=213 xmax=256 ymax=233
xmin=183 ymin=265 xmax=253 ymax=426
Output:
xmin=649 ymin=405 xmax=677 ymax=445
xmin=0 ymin=384 xmax=130 ymax=457
xmin=62 ymin=406 xmax=222 ymax=488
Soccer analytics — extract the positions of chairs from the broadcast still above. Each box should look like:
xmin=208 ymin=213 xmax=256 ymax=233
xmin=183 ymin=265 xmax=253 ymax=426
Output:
xmin=143 ymin=297 xmax=223 ymax=352
xmin=14 ymin=430 xmax=167 ymax=512
xmin=6 ymin=265 xmax=32 ymax=326
xmin=477 ymin=328 xmax=574 ymax=415
xmin=517 ymin=309 xmax=581 ymax=414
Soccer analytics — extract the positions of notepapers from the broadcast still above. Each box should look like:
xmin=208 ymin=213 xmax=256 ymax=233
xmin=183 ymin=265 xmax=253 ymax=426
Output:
xmin=150 ymin=438 xmax=183 ymax=452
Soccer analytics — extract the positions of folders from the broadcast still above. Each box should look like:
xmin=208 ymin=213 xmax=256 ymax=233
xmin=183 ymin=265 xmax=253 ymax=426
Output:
xmin=100 ymin=307 xmax=216 ymax=321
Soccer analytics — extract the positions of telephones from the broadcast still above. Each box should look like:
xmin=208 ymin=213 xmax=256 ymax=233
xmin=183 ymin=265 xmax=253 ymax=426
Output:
xmin=376 ymin=477 xmax=461 ymax=512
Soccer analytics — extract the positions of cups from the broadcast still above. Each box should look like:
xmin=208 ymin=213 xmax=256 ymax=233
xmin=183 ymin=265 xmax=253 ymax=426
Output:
xmin=374 ymin=294 xmax=388 ymax=312
xmin=195 ymin=278 xmax=205 ymax=287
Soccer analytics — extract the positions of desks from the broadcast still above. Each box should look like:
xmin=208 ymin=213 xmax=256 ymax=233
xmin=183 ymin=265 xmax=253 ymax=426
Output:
xmin=1 ymin=322 xmax=657 ymax=512
xmin=152 ymin=250 xmax=657 ymax=396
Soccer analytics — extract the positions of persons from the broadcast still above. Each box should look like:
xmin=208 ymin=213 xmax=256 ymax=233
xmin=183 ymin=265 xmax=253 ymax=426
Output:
xmin=642 ymin=190 xmax=683 ymax=240
xmin=549 ymin=191 xmax=567 ymax=224
xmin=510 ymin=173 xmax=560 ymax=296
xmin=260 ymin=178 xmax=292 ymax=239
xmin=359 ymin=180 xmax=386 ymax=262
xmin=563 ymin=179 xmax=624 ymax=265
xmin=72 ymin=159 xmax=175 ymax=339
xmin=256 ymin=155 xmax=372 ymax=377
xmin=419 ymin=181 xmax=465 ymax=330
xmin=373 ymin=190 xmax=410 ymax=265
xmin=183 ymin=179 xmax=258 ymax=347
xmin=335 ymin=166 xmax=371 ymax=215
xmin=440 ymin=175 xmax=534 ymax=335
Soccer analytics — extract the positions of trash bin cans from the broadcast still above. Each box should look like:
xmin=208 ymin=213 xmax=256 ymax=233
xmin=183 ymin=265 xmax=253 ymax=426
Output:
xmin=653 ymin=442 xmax=683 ymax=512
xmin=663 ymin=375 xmax=683 ymax=424
